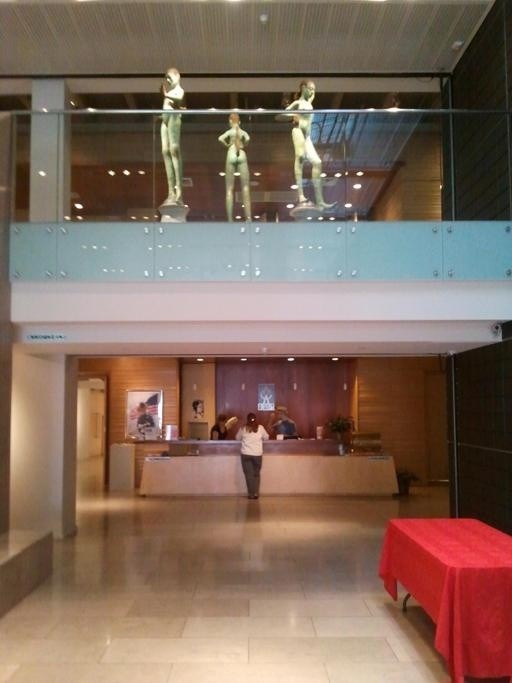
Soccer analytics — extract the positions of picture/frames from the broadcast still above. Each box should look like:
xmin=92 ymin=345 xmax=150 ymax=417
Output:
xmin=124 ymin=388 xmax=164 ymax=444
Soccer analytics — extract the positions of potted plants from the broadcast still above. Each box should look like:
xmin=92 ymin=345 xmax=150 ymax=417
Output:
xmin=393 ymin=464 xmax=421 ymax=494
xmin=327 ymin=415 xmax=353 ymax=441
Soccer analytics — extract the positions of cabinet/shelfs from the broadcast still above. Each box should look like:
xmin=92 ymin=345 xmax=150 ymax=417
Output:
xmin=109 ymin=440 xmax=135 ymax=491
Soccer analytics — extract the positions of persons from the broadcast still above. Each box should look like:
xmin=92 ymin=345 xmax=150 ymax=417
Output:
xmin=209 ymin=413 xmax=230 ymax=440
xmin=216 ymin=111 xmax=254 ymax=221
xmin=135 ymin=401 xmax=155 ymax=434
xmin=156 ymin=68 xmax=186 ymax=207
xmin=266 ymin=406 xmax=298 ymax=439
xmin=282 ymin=78 xmax=339 ymax=212
xmin=235 ymin=413 xmax=271 ymax=499
xmin=192 ymin=398 xmax=205 ymax=418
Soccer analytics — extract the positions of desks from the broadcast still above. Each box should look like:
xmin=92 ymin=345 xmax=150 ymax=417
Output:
xmin=378 ymin=516 xmax=512 ymax=682
